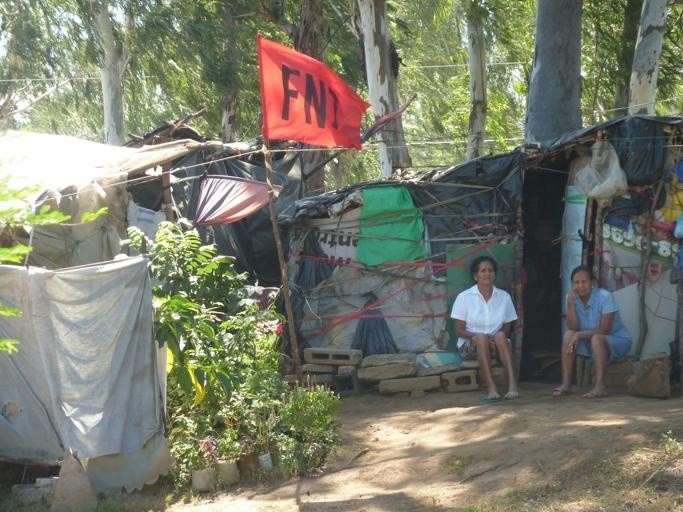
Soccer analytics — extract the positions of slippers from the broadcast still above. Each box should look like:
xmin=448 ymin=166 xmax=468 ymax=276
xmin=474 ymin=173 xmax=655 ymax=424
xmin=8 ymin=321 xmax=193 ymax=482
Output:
xmin=583 ymin=390 xmax=608 ymax=398
xmin=480 ymin=395 xmax=499 ymax=402
xmin=551 ymin=388 xmax=566 ymax=397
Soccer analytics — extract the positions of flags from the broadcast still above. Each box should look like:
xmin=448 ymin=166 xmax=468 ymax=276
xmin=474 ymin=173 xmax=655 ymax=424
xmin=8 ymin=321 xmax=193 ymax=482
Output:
xmin=253 ymin=32 xmax=370 ymax=154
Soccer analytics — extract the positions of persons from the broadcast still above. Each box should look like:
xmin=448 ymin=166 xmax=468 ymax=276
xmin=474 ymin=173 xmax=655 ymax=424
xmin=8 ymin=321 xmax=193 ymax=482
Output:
xmin=447 ymin=254 xmax=521 ymax=402
xmin=549 ymin=263 xmax=634 ymax=400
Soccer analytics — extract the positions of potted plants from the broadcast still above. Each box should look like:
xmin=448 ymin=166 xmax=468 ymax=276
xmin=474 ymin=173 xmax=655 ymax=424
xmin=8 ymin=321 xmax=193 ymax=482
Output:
xmin=121 ymin=219 xmax=344 ymax=496
